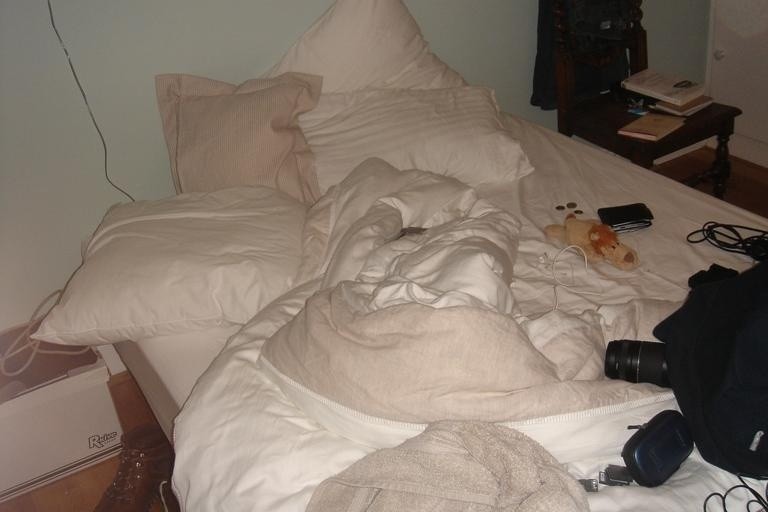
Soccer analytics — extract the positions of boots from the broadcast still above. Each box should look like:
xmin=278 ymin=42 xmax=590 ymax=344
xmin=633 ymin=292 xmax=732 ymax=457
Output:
xmin=94 ymin=424 xmax=169 ymax=512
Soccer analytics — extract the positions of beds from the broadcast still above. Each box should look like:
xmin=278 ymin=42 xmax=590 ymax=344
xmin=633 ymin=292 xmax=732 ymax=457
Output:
xmin=121 ymin=111 xmax=768 ymax=512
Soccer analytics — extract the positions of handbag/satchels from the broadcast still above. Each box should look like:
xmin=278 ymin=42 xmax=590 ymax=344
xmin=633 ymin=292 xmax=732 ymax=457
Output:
xmin=653 ymin=260 xmax=768 ymax=480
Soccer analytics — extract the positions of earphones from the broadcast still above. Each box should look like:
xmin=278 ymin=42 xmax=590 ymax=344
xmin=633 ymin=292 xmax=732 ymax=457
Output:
xmin=538 ymin=256 xmax=545 ymax=263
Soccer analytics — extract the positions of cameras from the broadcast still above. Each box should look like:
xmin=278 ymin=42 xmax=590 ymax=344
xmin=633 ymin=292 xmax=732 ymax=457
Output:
xmin=604 ymin=340 xmax=673 ymax=389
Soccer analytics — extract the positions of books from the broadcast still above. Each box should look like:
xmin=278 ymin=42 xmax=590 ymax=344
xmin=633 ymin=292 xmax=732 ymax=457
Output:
xmin=616 ymin=112 xmax=688 ymax=142
xmin=619 ymin=67 xmax=715 ymax=116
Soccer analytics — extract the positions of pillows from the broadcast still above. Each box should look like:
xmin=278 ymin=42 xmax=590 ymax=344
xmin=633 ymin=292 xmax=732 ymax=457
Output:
xmin=155 ymin=71 xmax=324 ymax=209
xmin=260 ymin=0 xmax=469 ymax=88
xmin=29 ymin=186 xmax=307 ymax=346
xmin=298 ymin=87 xmax=536 ymax=195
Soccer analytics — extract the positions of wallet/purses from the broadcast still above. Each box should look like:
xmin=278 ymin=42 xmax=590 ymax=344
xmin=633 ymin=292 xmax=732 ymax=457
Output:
xmin=621 ymin=410 xmax=694 ymax=487
xmin=598 ymin=203 xmax=654 ymax=234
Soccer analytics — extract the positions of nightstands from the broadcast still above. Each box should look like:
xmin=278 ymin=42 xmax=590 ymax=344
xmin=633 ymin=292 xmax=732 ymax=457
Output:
xmin=0 ymin=322 xmax=130 ymax=511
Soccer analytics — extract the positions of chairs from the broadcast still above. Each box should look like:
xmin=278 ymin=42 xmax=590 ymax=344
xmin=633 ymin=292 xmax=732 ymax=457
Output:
xmin=551 ymin=0 xmax=743 ymax=200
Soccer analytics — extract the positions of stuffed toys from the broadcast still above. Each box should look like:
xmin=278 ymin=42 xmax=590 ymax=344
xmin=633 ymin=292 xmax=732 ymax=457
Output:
xmin=546 ymin=212 xmax=641 ymax=273
